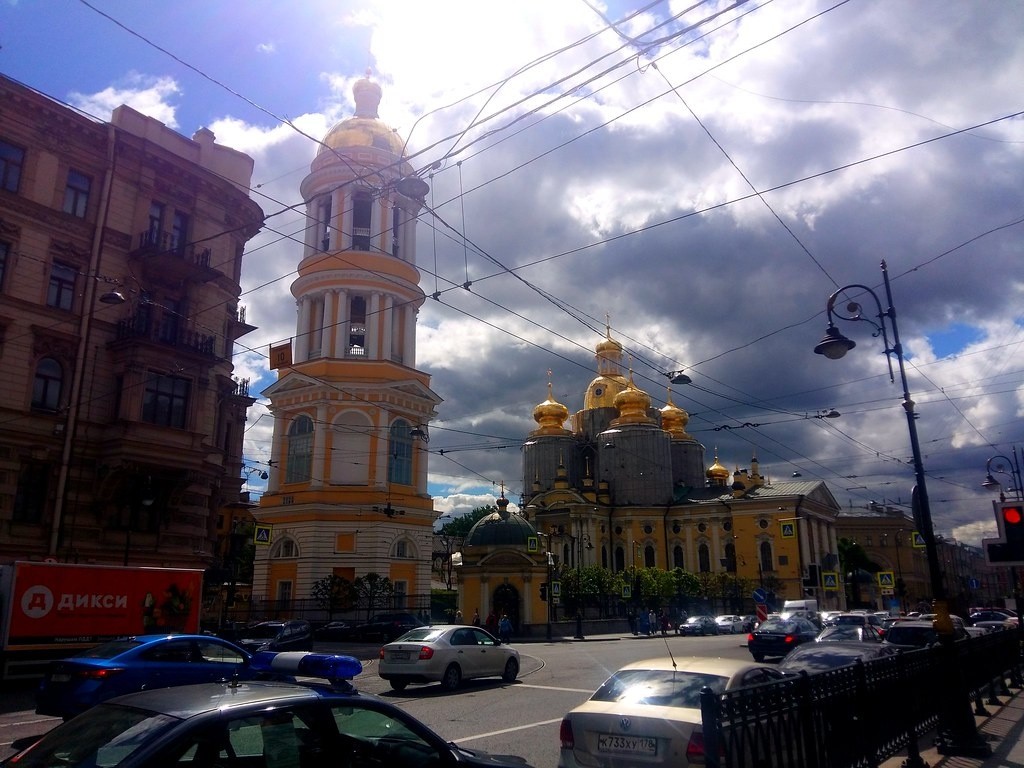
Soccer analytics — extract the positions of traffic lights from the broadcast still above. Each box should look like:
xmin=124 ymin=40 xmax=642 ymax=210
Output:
xmin=539 ymin=582 xmax=547 ymax=601
xmin=981 ymin=500 xmax=1024 ymax=569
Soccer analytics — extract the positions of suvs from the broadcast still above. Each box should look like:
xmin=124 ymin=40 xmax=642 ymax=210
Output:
xmin=237 ymin=620 xmax=314 ymax=656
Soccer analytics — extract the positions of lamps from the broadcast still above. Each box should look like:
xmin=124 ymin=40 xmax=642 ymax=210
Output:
xmin=409 ymin=427 xmax=424 ymax=436
xmin=670 ymin=372 xmax=692 ymax=383
xmin=100 ymin=290 xmax=124 ymax=305
xmin=826 ymin=408 xmax=841 ymax=418
xmin=261 ymin=471 xmax=268 ymax=479
xmin=792 ymin=470 xmax=802 ymax=478
xmin=906 ymin=458 xmax=915 ymax=464
xmin=397 ymin=174 xmax=430 ymax=199
xmin=603 ymin=441 xmax=616 ymax=449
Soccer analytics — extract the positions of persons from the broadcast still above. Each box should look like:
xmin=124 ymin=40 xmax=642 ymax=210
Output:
xmin=680 ymin=608 xmax=687 ymax=624
xmin=455 ymin=610 xmax=464 ymax=624
xmin=486 ymin=610 xmax=497 ymax=636
xmin=660 ymin=612 xmax=668 ymax=634
xmin=628 ymin=611 xmax=640 ymax=633
xmin=498 ymin=614 xmax=513 ymax=645
xmin=649 ymin=610 xmax=657 ymax=634
xmin=472 ymin=613 xmax=480 ymax=627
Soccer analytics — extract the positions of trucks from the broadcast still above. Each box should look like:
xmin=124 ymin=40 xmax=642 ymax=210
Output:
xmin=0 ymin=560 xmax=205 ymax=678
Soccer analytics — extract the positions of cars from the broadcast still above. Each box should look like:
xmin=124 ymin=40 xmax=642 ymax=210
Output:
xmin=377 ymin=624 xmax=521 ymax=692
xmin=558 ymin=655 xmax=797 ymax=768
xmin=715 ymin=599 xmax=1019 ymax=654
xmin=748 ymin=617 xmax=821 ymax=661
xmin=0 ymin=681 xmax=535 ymax=768
xmin=678 ymin=615 xmax=720 ymax=637
xmin=34 ymin=634 xmax=296 ymax=723
xmin=778 ymin=638 xmax=902 ymax=676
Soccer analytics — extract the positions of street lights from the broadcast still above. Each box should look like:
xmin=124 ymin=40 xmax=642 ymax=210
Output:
xmin=980 ymin=445 xmax=1024 ymax=502
xmin=574 ymin=529 xmax=595 ymax=641
xmin=812 ymin=257 xmax=992 ymax=759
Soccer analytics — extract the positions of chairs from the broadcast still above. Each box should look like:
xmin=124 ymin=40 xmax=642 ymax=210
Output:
xmin=189 ymin=643 xmax=206 ymax=664
xmin=466 ymin=633 xmax=478 ymax=646
xmin=454 ymin=634 xmax=464 ymax=645
xmin=260 ymin=716 xmax=306 ymax=768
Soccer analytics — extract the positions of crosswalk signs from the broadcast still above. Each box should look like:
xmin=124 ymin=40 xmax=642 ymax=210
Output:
xmin=254 ymin=525 xmax=272 ymax=546
xmin=822 ymin=571 xmax=838 ymax=591
xmin=621 ymin=585 xmax=631 ymax=598
xmin=878 ymin=572 xmax=895 ymax=588
xmin=781 ymin=522 xmax=796 ymax=539
xmin=528 ymin=537 xmax=538 ymax=552
xmin=912 ymin=531 xmax=927 ymax=548
xmin=552 ymin=581 xmax=561 ymax=597
